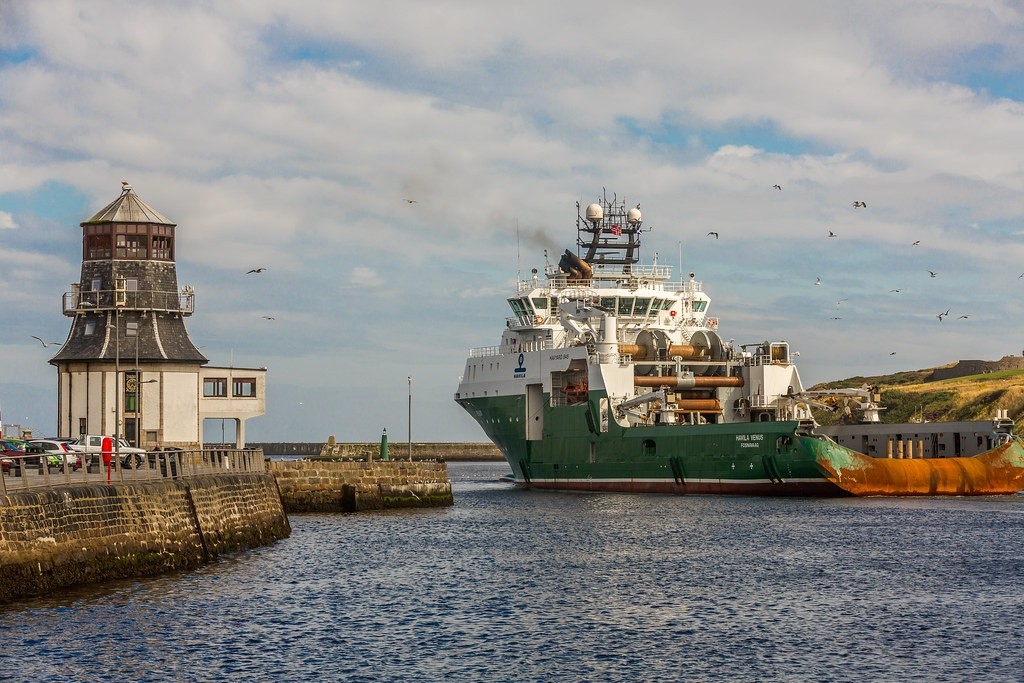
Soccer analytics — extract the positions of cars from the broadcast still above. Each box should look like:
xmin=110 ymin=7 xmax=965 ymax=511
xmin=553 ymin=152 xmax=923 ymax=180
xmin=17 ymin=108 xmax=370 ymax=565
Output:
xmin=0 ymin=441 xmax=26 ymax=472
xmin=26 ymin=440 xmax=77 ymax=471
xmin=0 ymin=439 xmax=45 ymax=469
xmin=69 ymin=435 xmax=146 ymax=468
xmin=42 ymin=437 xmax=78 ymax=451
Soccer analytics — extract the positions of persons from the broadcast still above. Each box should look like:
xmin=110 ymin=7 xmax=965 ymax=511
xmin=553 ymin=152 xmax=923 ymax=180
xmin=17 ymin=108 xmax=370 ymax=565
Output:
xmin=153 ymin=444 xmax=162 ymax=451
xmin=119 ymin=434 xmax=124 ymax=439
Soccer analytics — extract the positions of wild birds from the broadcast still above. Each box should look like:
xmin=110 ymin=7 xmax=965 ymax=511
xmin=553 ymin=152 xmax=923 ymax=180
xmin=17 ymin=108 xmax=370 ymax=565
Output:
xmin=27 ymin=168 xmax=969 ymax=356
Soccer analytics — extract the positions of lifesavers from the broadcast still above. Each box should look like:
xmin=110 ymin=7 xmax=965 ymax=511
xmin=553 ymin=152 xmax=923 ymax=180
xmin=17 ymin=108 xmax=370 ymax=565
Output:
xmin=535 ymin=316 xmax=544 ymax=324
xmin=709 ymin=318 xmax=717 ymax=327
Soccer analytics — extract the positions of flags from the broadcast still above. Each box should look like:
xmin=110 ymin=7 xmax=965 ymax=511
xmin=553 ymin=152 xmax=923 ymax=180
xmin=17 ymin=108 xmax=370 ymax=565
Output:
xmin=611 ymin=225 xmax=622 ymax=236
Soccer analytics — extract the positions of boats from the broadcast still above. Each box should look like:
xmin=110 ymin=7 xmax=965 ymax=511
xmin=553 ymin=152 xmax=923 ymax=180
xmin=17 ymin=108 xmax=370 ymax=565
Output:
xmin=455 ymin=186 xmax=1024 ymax=497
